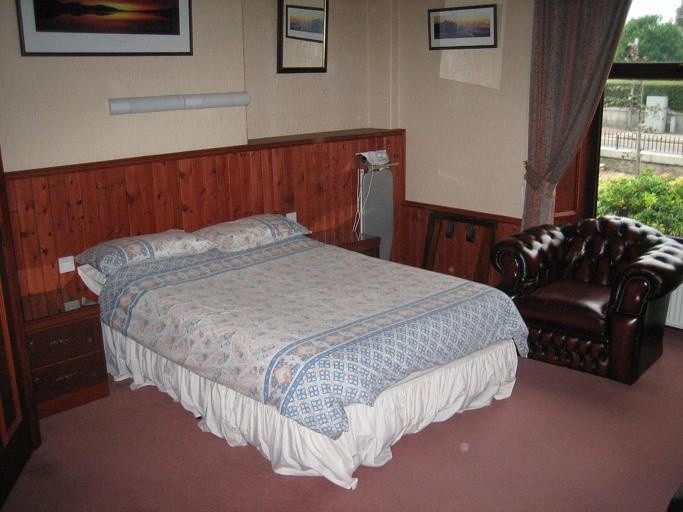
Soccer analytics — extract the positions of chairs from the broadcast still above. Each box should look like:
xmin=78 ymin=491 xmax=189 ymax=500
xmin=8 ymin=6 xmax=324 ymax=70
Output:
xmin=496 ymin=213 xmax=683 ymax=387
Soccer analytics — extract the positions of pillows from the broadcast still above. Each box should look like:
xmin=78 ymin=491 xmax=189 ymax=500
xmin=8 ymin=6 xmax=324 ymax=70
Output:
xmin=75 ymin=228 xmax=216 ymax=275
xmin=199 ymin=212 xmax=314 ymax=252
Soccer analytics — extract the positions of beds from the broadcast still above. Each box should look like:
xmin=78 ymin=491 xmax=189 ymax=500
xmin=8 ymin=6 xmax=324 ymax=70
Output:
xmin=76 ymin=234 xmax=529 ymax=489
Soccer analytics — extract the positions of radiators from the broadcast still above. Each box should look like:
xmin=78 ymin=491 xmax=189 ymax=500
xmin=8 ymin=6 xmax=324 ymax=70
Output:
xmin=666 ymin=284 xmax=683 ymax=329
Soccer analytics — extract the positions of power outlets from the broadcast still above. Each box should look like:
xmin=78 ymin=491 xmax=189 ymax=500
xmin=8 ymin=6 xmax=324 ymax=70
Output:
xmin=286 ymin=212 xmax=297 ymax=223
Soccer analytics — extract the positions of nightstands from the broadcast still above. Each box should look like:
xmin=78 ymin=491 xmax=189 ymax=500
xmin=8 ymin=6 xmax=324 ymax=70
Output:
xmin=20 ymin=297 xmax=110 ymax=420
xmin=311 ymin=229 xmax=381 ymax=258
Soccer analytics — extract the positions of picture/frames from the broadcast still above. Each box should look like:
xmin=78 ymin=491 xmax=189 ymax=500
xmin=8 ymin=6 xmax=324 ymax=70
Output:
xmin=15 ymin=0 xmax=193 ymax=58
xmin=428 ymin=4 xmax=496 ymax=50
xmin=278 ymin=0 xmax=329 ymax=74
